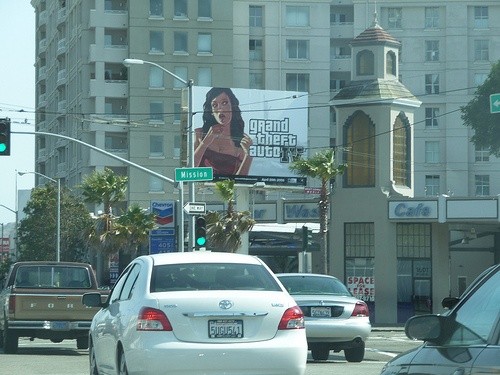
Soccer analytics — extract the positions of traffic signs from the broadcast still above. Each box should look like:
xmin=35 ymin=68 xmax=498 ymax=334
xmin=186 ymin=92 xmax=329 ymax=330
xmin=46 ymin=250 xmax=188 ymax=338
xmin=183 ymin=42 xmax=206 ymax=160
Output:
xmin=175 ymin=167 xmax=214 ymax=181
xmin=183 ymin=202 xmax=207 ymax=215
xmin=490 ymin=93 xmax=500 ymax=113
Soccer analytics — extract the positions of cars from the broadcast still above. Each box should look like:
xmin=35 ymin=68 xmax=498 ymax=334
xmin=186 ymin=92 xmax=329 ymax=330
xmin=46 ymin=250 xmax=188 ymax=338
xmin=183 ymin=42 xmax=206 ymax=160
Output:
xmin=81 ymin=251 xmax=310 ymax=375
xmin=274 ymin=272 xmax=372 ymax=362
xmin=380 ymin=264 xmax=500 ymax=375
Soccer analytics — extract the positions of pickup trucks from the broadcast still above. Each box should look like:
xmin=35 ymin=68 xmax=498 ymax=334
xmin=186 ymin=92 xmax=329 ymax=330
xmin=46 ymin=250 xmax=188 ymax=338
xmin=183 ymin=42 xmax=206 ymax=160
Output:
xmin=0 ymin=260 xmax=105 ymax=353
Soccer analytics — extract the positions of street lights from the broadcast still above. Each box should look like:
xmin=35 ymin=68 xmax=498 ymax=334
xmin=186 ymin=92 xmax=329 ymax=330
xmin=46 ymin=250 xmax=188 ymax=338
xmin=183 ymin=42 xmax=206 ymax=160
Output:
xmin=18 ymin=170 xmax=61 ymax=262
xmin=123 ymin=58 xmax=197 ymax=253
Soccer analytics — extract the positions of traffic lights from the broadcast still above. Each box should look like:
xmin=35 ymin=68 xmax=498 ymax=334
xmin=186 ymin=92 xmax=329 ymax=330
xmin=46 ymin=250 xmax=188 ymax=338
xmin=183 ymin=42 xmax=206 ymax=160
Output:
xmin=195 ymin=216 xmax=208 ymax=248
xmin=0 ymin=117 xmax=12 ymax=156
